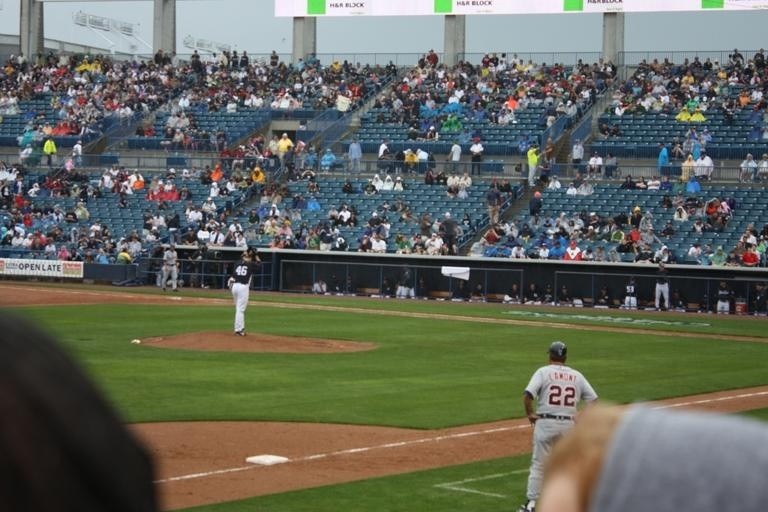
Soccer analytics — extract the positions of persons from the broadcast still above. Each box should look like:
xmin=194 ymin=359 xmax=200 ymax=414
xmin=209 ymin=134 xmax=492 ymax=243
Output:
xmin=0 ymin=47 xmax=768 ymax=314
xmin=228 ymin=251 xmax=261 ymax=336
xmin=535 ymin=399 xmax=768 ymax=512
xmin=518 ymin=341 xmax=598 ymax=512
xmin=1 ymin=311 xmax=161 ymax=512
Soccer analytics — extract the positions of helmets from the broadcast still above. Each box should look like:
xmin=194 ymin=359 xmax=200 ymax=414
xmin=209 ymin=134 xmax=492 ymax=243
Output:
xmin=545 ymin=339 xmax=569 ymax=359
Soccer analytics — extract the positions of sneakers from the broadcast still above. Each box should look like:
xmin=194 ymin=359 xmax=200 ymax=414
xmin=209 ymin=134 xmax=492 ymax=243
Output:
xmin=234 ymin=328 xmax=246 ymax=336
xmin=516 ymin=498 xmax=537 ymax=512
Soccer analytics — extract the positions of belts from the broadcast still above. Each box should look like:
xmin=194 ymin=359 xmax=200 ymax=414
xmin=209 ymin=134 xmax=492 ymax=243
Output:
xmin=235 ymin=280 xmax=248 ymax=285
xmin=537 ymin=413 xmax=575 ymax=419
xmin=167 ymin=264 xmax=177 ymax=267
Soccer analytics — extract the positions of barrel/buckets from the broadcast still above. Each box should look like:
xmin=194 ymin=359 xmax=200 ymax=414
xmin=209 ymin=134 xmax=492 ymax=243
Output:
xmin=735 ymin=297 xmax=746 ymax=313
